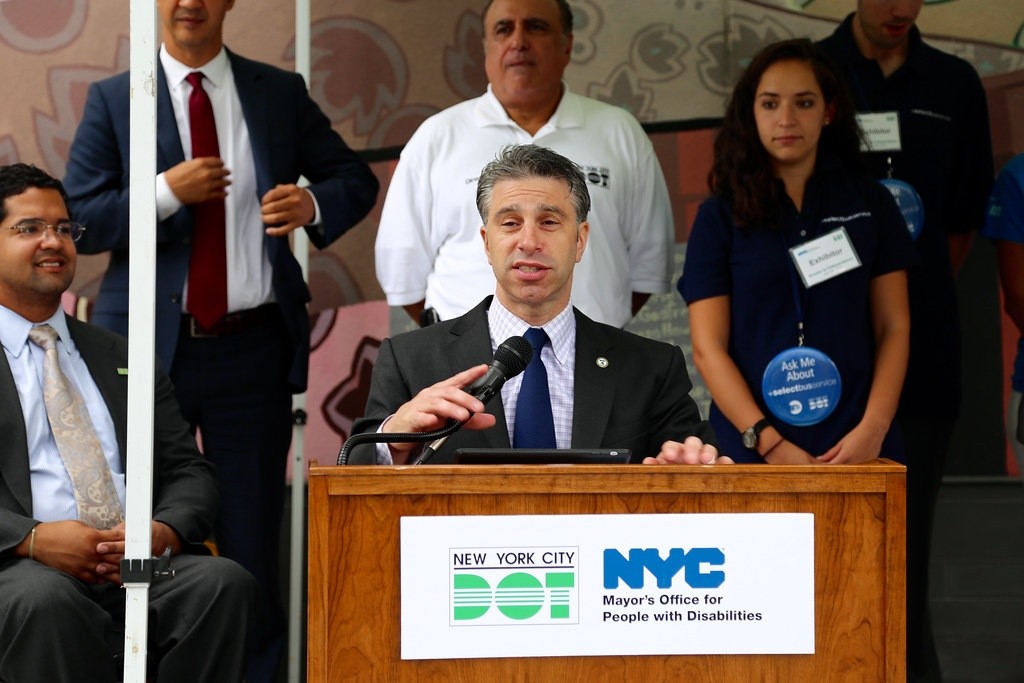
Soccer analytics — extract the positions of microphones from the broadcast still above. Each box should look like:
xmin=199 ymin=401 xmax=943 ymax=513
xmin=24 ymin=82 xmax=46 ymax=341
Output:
xmin=413 ymin=336 xmax=534 ymax=468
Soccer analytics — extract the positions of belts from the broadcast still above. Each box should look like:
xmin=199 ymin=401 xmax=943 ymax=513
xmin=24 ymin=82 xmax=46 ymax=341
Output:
xmin=181 ymin=308 xmax=282 ymax=338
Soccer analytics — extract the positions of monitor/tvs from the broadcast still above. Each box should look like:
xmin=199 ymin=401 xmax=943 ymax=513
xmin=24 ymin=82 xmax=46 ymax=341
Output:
xmin=454 ymin=449 xmax=632 ymax=464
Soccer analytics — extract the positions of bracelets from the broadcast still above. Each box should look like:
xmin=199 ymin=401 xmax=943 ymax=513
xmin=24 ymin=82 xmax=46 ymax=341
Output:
xmin=761 ymin=438 xmax=785 ymax=459
xmin=28 ymin=525 xmax=36 ymax=560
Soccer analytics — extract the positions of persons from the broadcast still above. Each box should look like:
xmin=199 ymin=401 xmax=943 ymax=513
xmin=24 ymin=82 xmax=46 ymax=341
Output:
xmin=981 ymin=150 xmax=1024 ymax=447
xmin=813 ymin=0 xmax=995 ymax=683
xmin=374 ymin=0 xmax=675 ymax=330
xmin=0 ymin=162 xmax=259 ymax=683
xmin=61 ymin=0 xmax=379 ymax=683
xmin=335 ymin=144 xmax=737 ymax=465
xmin=677 ymin=38 xmax=920 ymax=465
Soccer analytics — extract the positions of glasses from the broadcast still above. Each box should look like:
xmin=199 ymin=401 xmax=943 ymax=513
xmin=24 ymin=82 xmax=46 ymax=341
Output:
xmin=0 ymin=220 xmax=85 ymax=242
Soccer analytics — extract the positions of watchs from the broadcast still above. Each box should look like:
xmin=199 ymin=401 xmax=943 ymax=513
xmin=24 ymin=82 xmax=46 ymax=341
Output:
xmin=741 ymin=417 xmax=772 ymax=450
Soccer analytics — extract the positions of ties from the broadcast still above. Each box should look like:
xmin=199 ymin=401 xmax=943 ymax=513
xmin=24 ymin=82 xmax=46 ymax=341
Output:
xmin=183 ymin=71 xmax=228 ymax=330
xmin=513 ymin=328 xmax=558 ymax=451
xmin=27 ymin=324 xmax=124 ymax=529
